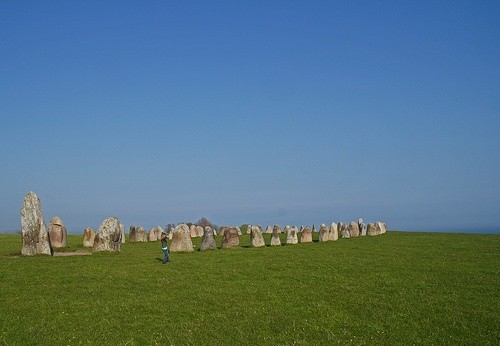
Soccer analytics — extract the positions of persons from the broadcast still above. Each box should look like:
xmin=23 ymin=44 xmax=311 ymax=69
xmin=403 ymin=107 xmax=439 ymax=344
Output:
xmin=161 ymin=236 xmax=170 ymax=264
